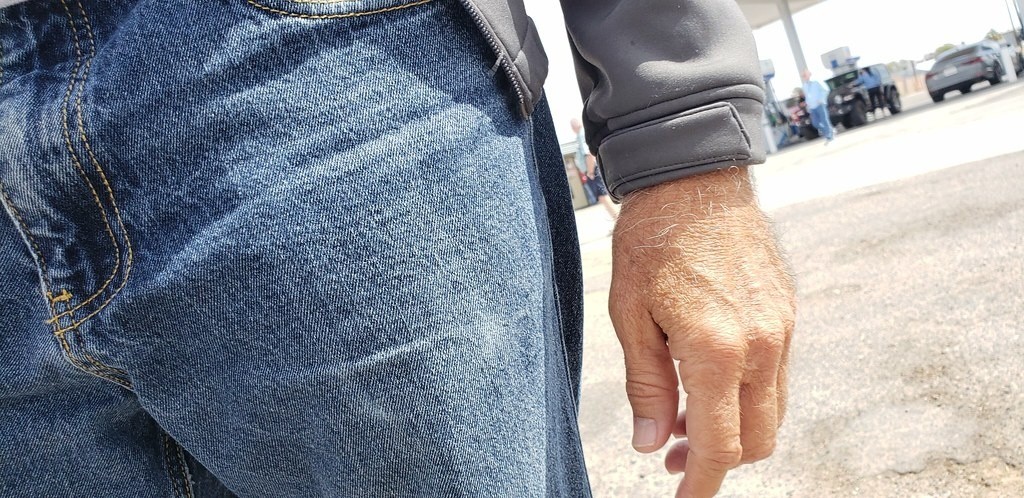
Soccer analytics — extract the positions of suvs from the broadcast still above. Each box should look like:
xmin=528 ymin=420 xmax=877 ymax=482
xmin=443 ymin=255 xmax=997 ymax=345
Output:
xmin=795 ymin=62 xmax=904 ymax=140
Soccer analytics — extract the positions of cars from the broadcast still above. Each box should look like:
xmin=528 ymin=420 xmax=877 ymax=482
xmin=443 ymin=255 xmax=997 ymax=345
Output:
xmin=924 ymin=41 xmax=1005 ymax=102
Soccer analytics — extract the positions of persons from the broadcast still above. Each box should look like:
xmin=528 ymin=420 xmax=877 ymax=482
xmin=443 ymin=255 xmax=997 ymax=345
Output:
xmin=0 ymin=0 xmax=798 ymax=498
xmin=849 ymin=66 xmax=886 ymax=120
xmin=801 ymin=69 xmax=834 ymax=146
xmin=570 ymin=116 xmax=619 ymax=219
